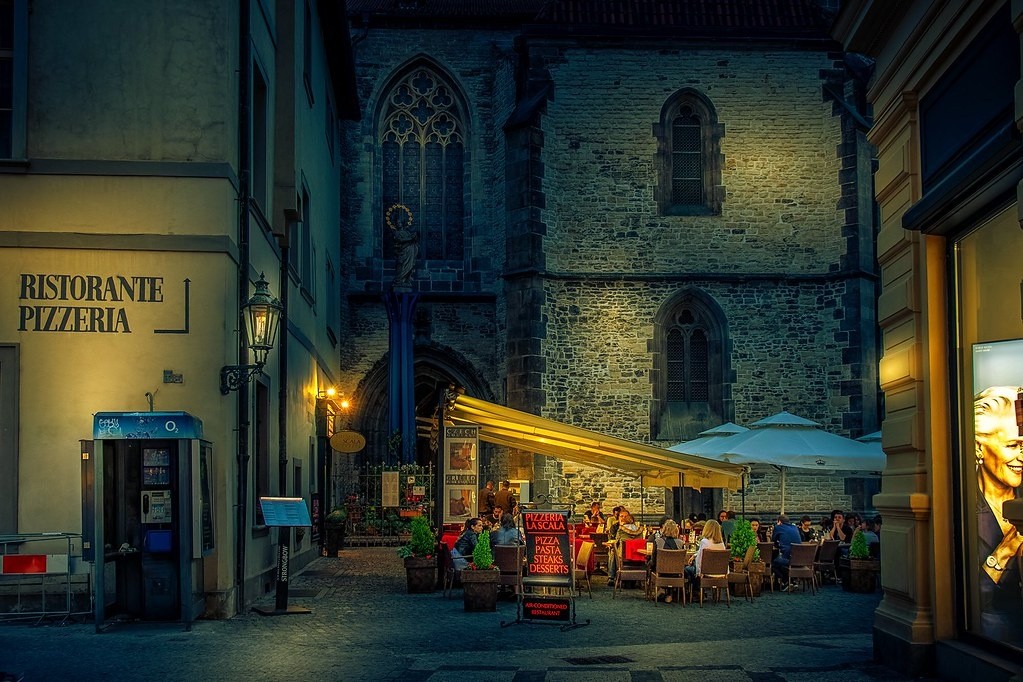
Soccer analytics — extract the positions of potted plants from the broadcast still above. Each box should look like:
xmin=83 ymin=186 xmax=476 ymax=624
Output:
xmin=460 ymin=530 xmax=500 ymax=612
xmin=325 ymin=506 xmax=347 ymax=559
xmin=842 ymin=530 xmax=875 ymax=592
xmin=395 ymin=515 xmax=437 ymax=595
xmin=730 ymin=516 xmax=765 ymax=598
xmin=358 ymin=511 xmax=379 ymax=535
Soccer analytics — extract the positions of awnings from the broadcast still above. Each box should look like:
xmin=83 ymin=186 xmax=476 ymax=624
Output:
xmin=429 ymin=392 xmax=749 ymax=494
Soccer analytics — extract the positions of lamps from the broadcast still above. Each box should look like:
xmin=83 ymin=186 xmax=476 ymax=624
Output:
xmin=219 ymin=271 xmax=285 ymax=395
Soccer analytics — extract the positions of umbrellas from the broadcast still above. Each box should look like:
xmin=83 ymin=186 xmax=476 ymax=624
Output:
xmin=665 ymin=410 xmax=887 ymax=515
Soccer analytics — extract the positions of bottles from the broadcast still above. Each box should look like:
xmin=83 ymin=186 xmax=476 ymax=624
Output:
xmin=682 ymin=529 xmax=702 ymax=551
xmin=645 ymin=526 xmax=660 ymax=541
xmin=814 ymin=527 xmax=830 ymax=543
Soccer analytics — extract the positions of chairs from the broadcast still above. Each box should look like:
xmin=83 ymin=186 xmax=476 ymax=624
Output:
xmin=574 ymin=524 xmax=881 ymax=608
xmin=491 ymin=544 xmax=522 ymax=595
xmin=440 ymin=541 xmax=474 ymax=601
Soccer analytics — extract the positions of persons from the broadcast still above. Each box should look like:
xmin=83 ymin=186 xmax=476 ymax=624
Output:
xmin=455 ymin=481 xmax=882 ymax=602
xmin=975 ymin=385 xmax=1023 ymax=641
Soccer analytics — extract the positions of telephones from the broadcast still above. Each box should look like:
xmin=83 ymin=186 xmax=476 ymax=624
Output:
xmin=141 ymin=490 xmax=172 ymax=524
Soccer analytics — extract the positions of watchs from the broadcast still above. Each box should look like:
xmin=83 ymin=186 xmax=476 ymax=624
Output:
xmin=986 ymin=555 xmax=1005 ymax=571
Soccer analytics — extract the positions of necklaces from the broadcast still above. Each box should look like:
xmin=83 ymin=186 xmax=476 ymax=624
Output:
xmin=989 ymin=502 xmax=1007 ymax=523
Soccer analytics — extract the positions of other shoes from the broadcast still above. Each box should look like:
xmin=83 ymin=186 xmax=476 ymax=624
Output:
xmin=607 ymin=579 xmax=614 ymax=586
xmin=665 ymin=595 xmax=672 ymax=603
xmin=792 ymin=581 xmax=798 ymax=589
xmin=780 ymin=583 xmax=793 ymax=591
